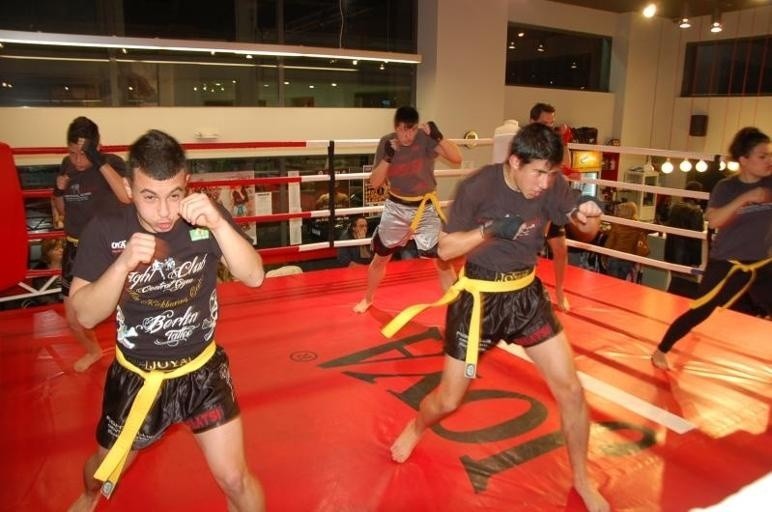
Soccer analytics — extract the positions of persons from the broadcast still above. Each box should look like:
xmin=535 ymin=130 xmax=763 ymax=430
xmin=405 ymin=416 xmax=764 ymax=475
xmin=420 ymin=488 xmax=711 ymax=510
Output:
xmin=353 ymin=107 xmax=463 ymax=314
xmin=52 ymin=116 xmax=134 ymax=373
xmin=33 ymin=239 xmax=66 ymax=306
xmin=338 ymin=217 xmax=377 ymax=266
xmin=651 ymin=128 xmax=771 ymax=370
xmin=529 ymin=103 xmax=581 ymax=312
xmin=389 ymin=122 xmax=610 ymax=511
xmin=67 ymin=131 xmax=266 ymax=512
xmin=664 ymin=181 xmax=704 ymax=292
xmin=600 ymin=202 xmax=658 ymax=280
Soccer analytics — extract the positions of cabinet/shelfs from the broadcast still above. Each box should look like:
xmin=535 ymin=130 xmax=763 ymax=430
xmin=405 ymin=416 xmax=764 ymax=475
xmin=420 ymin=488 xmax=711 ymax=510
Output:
xmin=624 ymin=170 xmax=659 ymax=224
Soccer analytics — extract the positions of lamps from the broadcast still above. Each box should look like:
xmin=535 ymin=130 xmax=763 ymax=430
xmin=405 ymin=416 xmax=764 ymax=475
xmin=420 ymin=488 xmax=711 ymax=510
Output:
xmin=676 ymin=2 xmax=723 ymax=32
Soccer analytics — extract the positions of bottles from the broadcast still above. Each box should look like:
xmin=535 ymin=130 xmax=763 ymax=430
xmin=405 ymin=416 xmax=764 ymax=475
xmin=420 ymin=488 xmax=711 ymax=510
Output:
xmin=571 ymin=180 xmax=587 ymax=192
xmin=600 ymin=221 xmax=611 ymax=231
xmin=603 ymin=187 xmax=617 ymax=203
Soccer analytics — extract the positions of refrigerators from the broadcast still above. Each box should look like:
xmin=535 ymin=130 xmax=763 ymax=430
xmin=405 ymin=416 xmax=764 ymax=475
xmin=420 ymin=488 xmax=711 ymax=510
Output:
xmin=568 ymin=150 xmax=603 ymax=203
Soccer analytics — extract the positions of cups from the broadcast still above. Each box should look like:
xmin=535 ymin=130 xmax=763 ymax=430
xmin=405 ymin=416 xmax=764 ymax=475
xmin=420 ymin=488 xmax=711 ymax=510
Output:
xmin=658 ymin=231 xmax=663 ymax=237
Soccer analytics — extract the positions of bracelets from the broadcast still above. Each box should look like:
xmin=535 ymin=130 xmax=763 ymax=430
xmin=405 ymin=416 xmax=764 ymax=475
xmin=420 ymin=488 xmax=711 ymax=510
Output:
xmin=479 ymin=225 xmax=485 ymax=242
xmin=55 ymin=185 xmax=63 ymax=198
